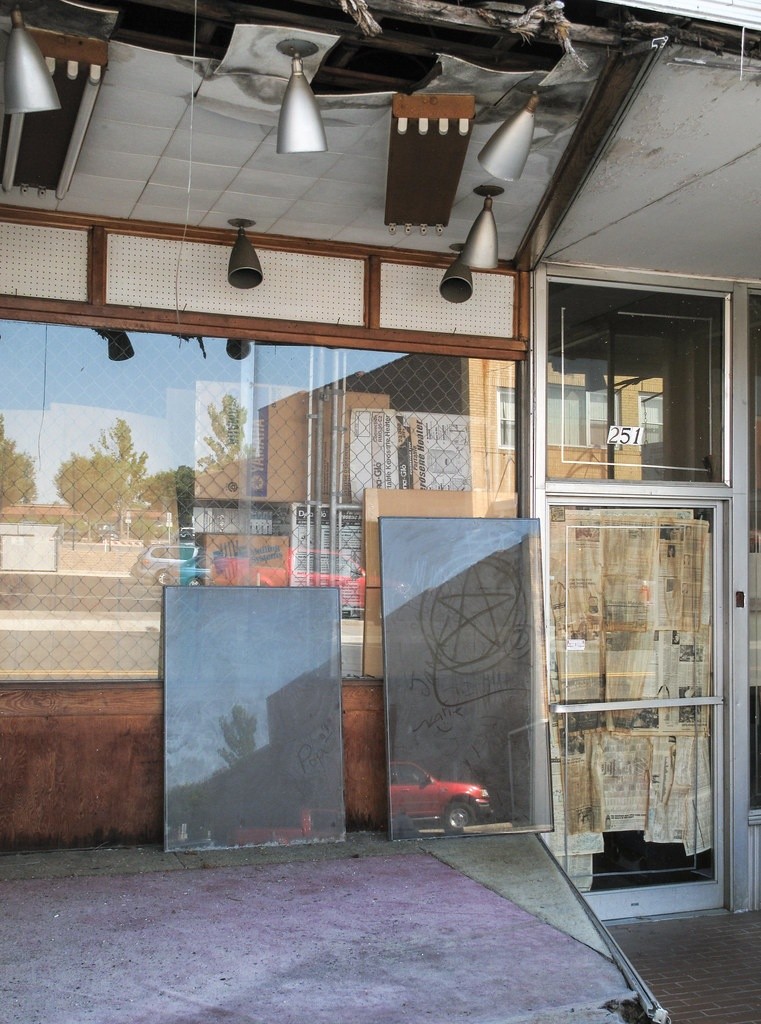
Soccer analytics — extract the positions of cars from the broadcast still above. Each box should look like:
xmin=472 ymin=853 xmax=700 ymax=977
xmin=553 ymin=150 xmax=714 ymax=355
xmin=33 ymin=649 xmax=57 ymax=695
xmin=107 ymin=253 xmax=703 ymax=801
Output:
xmin=166 ymin=555 xmax=211 ymax=585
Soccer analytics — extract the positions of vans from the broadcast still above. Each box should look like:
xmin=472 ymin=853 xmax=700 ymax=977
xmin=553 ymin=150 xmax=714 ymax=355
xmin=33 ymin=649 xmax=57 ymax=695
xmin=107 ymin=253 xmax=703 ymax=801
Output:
xmin=129 ymin=545 xmax=203 ymax=586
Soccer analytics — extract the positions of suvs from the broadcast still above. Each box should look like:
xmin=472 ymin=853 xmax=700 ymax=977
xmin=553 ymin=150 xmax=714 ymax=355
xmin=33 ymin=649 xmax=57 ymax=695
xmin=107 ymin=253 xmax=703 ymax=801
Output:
xmin=92 ymin=522 xmax=120 ymax=545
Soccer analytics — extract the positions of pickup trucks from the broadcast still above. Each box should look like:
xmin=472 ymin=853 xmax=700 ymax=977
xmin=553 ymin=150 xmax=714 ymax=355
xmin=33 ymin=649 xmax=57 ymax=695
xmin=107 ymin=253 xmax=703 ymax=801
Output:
xmin=223 ymin=807 xmax=346 ymax=846
xmin=389 ymin=760 xmax=492 ymax=845
xmin=216 ymin=544 xmax=368 ymax=618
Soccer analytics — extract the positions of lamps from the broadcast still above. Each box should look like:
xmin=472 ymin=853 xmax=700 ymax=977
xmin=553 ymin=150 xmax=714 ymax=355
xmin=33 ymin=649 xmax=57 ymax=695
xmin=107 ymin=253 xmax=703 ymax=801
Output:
xmin=5 ymin=1 xmax=62 ymax=114
xmin=226 ymin=340 xmax=252 ymax=360
xmin=275 ymin=51 xmax=328 ymax=153
xmin=439 ymin=253 xmax=473 ymax=303
xmin=228 ymin=226 xmax=263 ymax=290
xmin=477 ymin=92 xmax=541 ymax=182
xmin=461 ymin=194 xmax=499 ymax=269
xmin=108 ymin=332 xmax=134 ymax=361
